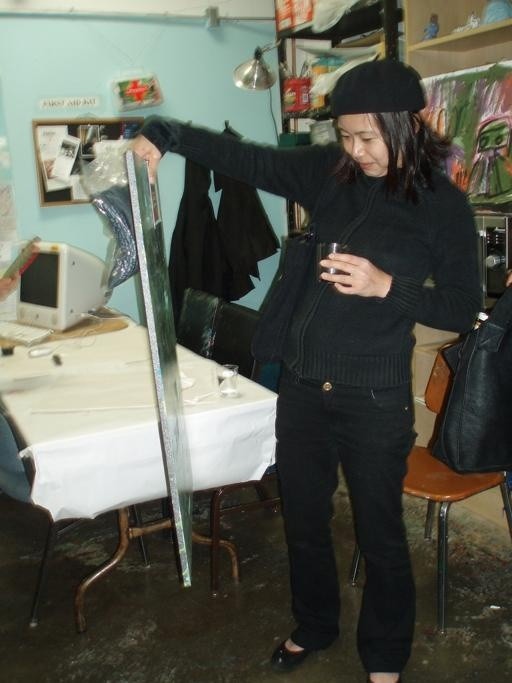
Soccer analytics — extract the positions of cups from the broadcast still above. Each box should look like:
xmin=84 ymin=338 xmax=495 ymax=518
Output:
xmin=317 ymin=242 xmax=351 ymax=285
xmin=216 ymin=364 xmax=239 ymax=398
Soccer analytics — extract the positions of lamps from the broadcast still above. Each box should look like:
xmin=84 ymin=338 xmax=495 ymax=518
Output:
xmin=230 ymin=34 xmax=284 ymax=92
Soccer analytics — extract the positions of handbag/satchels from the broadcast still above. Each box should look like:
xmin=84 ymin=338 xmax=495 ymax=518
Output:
xmin=426 ymin=284 xmax=512 ymax=474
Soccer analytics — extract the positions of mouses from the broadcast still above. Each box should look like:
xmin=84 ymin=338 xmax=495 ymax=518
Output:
xmin=28 ymin=346 xmax=52 ymax=356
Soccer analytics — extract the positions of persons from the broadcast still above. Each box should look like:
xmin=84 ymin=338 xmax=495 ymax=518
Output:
xmin=124 ymin=59 xmax=485 ymax=683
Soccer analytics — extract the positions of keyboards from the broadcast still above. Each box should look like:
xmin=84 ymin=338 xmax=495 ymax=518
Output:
xmin=0 ymin=321 xmax=52 ymax=346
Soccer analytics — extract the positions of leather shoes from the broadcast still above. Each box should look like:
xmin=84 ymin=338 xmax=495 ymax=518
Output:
xmin=270 ymin=619 xmax=341 ymax=675
xmin=366 ymin=671 xmax=401 ymax=683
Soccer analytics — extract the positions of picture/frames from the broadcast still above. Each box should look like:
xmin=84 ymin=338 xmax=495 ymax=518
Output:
xmin=33 ymin=119 xmax=152 ymax=207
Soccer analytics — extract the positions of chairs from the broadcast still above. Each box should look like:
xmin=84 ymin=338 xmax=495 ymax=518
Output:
xmin=0 ymin=412 xmax=83 ymax=630
xmin=179 ymin=290 xmax=278 ymax=599
xmin=347 ymin=338 xmax=512 ymax=635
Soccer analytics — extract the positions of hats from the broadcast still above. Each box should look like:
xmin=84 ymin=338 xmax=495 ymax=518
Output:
xmin=329 ymin=59 xmax=427 ymax=116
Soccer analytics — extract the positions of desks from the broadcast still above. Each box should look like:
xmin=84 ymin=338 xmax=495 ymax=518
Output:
xmin=0 ymin=314 xmax=281 ymax=638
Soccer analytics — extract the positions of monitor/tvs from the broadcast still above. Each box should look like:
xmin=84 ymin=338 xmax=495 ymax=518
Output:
xmin=15 ymin=240 xmax=114 ymax=331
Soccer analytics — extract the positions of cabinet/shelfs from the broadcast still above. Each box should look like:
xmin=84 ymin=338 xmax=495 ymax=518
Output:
xmin=407 ymin=1 xmax=512 ymax=526
xmin=273 ymin=0 xmax=406 ymax=230
